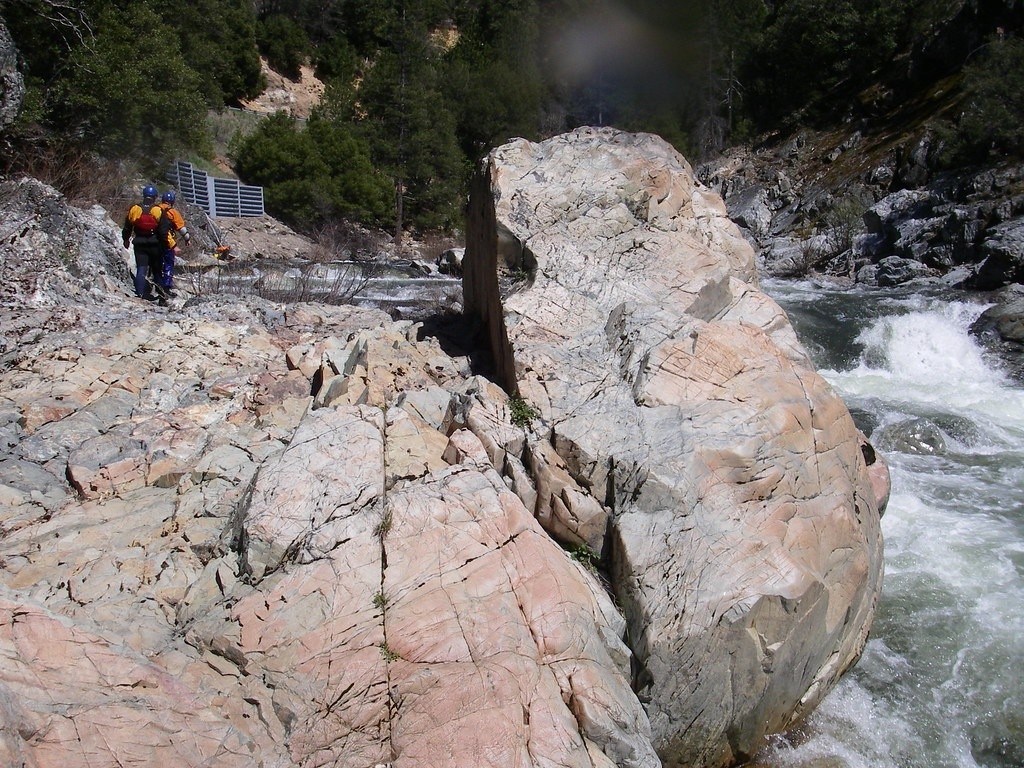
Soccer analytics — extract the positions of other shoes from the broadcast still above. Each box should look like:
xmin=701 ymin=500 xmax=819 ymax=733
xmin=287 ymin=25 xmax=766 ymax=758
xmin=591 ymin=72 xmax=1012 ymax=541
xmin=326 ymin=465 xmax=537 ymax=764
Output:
xmin=159 ymin=300 xmax=168 ymax=308
xmin=164 ymin=288 xmax=177 ymax=298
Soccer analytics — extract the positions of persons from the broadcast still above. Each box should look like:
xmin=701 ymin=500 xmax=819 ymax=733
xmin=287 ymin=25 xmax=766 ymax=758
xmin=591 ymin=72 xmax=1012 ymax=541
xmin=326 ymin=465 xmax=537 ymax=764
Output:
xmin=122 ymin=185 xmax=190 ymax=307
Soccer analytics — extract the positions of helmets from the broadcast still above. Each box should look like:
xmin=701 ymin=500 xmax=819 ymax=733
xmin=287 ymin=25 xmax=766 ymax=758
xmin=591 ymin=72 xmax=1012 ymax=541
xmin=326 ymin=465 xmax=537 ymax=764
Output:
xmin=143 ymin=186 xmax=158 ymax=197
xmin=162 ymin=192 xmax=176 ymax=204
